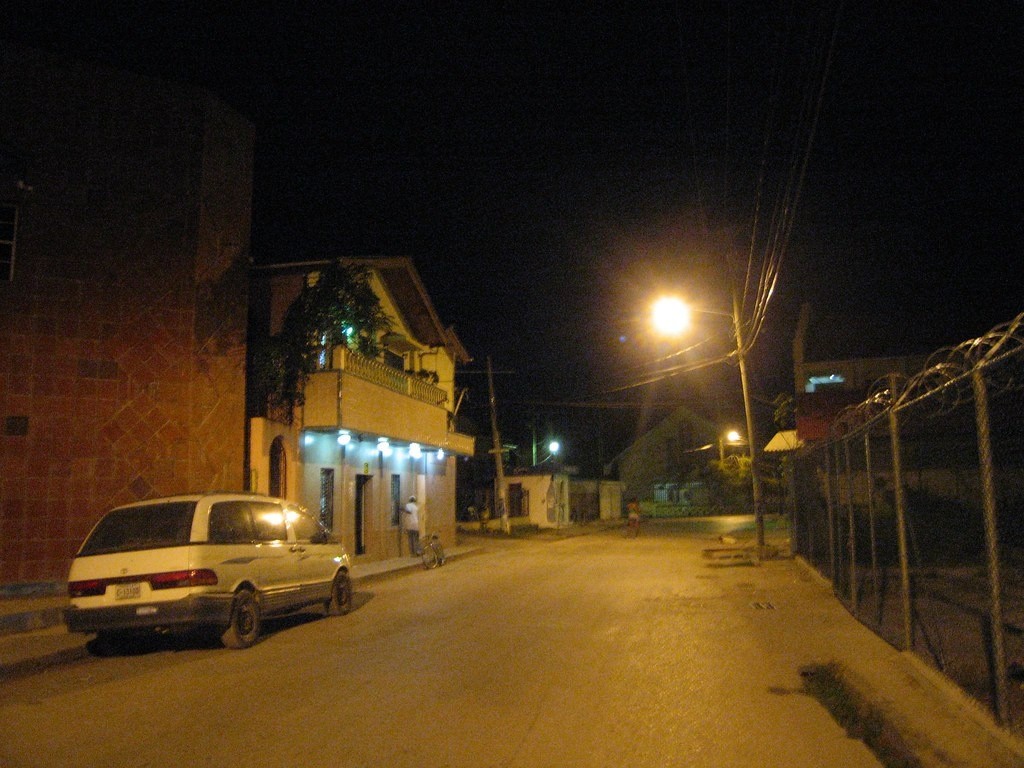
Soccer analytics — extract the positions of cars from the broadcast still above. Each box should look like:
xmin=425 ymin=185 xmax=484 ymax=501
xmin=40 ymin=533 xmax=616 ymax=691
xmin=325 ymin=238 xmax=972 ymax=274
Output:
xmin=66 ymin=488 xmax=354 ymax=649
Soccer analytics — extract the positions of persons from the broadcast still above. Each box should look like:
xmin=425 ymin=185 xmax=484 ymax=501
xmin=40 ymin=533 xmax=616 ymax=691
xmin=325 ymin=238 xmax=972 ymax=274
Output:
xmin=468 ymin=504 xmax=488 ymax=532
xmin=399 ymin=496 xmax=421 ymax=558
xmin=626 ymin=498 xmax=641 ymax=537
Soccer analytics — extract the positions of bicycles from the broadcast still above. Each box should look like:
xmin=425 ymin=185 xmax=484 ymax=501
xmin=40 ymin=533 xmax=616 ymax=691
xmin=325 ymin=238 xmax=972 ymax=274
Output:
xmin=420 ymin=529 xmax=446 ymax=570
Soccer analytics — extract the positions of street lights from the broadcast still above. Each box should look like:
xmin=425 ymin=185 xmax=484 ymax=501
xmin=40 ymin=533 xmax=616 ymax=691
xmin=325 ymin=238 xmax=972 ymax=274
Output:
xmin=658 ymin=295 xmax=768 ymax=547
